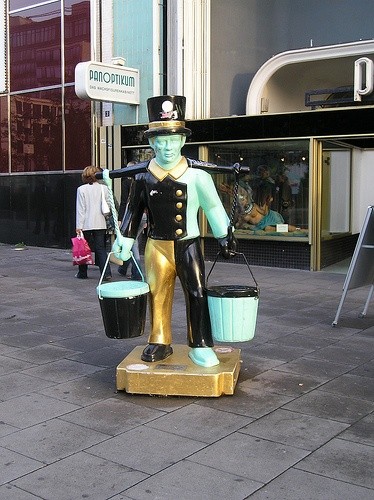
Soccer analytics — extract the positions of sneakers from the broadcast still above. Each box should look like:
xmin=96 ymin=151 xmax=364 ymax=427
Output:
xmin=75 ymin=274 xmax=87 ymax=279
xmin=127 ymin=275 xmax=142 ymax=280
xmin=102 ymin=274 xmax=112 ymax=281
xmin=120 ymin=274 xmax=126 ymax=277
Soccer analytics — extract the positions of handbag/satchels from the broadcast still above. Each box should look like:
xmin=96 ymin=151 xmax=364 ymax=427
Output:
xmin=71 ymin=229 xmax=95 ymax=265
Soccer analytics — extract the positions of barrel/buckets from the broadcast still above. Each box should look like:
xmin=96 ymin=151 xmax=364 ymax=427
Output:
xmin=95 ymin=251 xmax=150 ymax=339
xmin=205 ymin=250 xmax=260 ymax=343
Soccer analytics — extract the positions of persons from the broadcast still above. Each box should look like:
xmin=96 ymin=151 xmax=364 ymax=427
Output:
xmin=112 ymin=95 xmax=236 ymax=368
xmin=74 ymin=166 xmax=144 ymax=281
xmin=231 ymin=191 xmax=308 ymax=233
